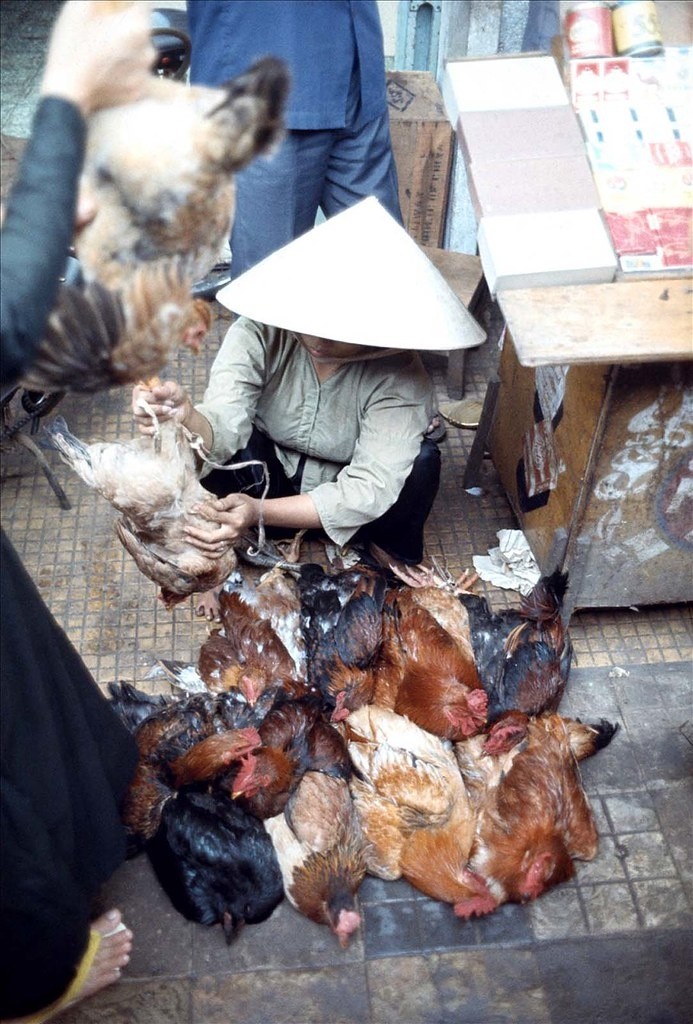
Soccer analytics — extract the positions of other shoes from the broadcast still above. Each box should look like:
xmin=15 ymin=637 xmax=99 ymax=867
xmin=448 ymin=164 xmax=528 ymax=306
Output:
xmin=357 ymin=546 xmax=410 ymax=586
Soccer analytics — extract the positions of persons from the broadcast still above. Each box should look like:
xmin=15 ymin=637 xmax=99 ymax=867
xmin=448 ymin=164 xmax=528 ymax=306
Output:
xmin=131 ymin=195 xmax=490 ymax=565
xmin=0 ymin=0 xmax=160 ymax=1024
xmin=185 ymin=0 xmax=406 ymax=286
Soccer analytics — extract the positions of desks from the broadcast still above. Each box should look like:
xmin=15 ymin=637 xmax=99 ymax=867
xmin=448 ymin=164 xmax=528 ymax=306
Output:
xmin=462 ymin=303 xmax=693 ymax=635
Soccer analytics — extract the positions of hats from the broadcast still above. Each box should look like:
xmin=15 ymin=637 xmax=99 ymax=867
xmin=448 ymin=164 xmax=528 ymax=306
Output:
xmin=215 ymin=196 xmax=487 ymax=351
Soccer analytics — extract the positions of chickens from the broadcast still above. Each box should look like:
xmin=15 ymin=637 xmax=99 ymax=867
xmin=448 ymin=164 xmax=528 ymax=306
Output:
xmin=469 ymin=709 xmax=621 ymax=907
xmin=21 ymin=1 xmax=288 ymax=390
xmin=40 ymin=377 xmax=239 ymax=610
xmin=103 ymin=528 xmax=573 ymax=947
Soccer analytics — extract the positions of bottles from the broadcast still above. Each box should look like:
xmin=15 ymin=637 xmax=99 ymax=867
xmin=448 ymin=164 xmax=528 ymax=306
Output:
xmin=612 ymin=1 xmax=663 ymax=58
xmin=565 ymin=2 xmax=615 ymax=58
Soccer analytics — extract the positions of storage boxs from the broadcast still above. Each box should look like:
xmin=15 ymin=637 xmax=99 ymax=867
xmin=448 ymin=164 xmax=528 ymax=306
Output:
xmin=384 ymin=70 xmax=451 ymax=251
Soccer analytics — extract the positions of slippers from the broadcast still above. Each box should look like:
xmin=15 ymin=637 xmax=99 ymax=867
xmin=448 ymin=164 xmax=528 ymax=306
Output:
xmin=0 ymin=922 xmax=126 ymax=1024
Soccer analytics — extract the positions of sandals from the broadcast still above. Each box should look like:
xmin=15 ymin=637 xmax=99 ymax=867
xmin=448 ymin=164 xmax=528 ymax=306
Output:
xmin=424 ymin=413 xmax=446 ymax=441
